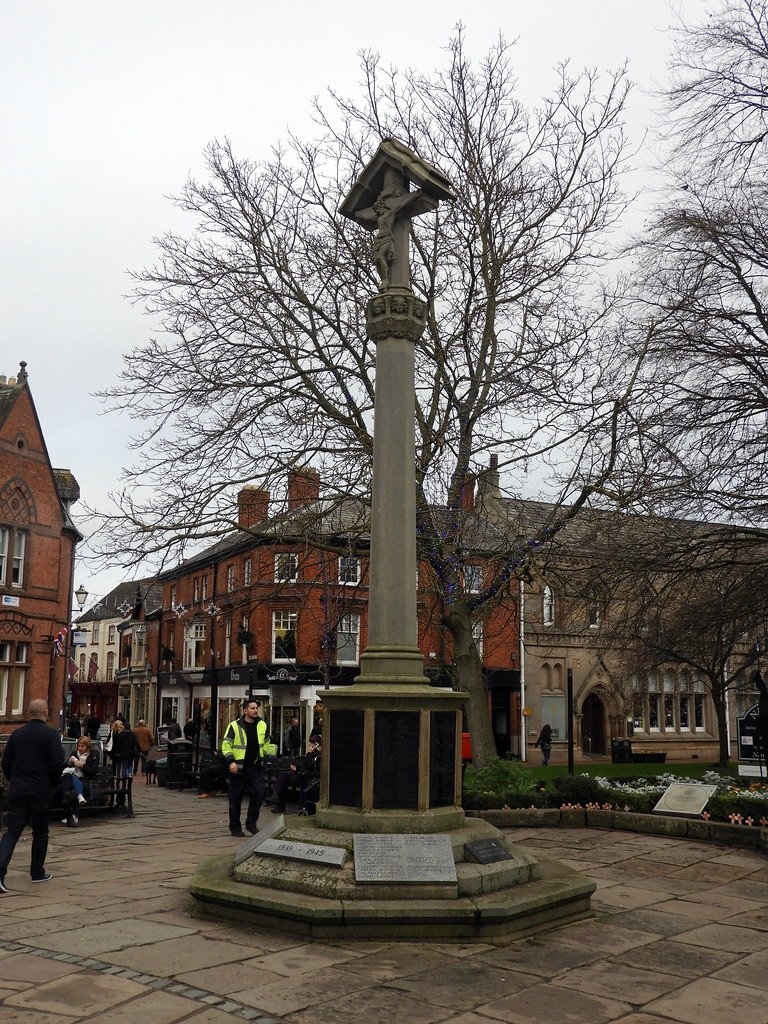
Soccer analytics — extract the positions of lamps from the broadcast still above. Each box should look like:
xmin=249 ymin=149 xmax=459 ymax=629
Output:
xmin=71 ymin=584 xmax=88 ymax=613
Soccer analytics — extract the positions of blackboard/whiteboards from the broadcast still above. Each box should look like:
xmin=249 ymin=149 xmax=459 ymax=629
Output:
xmin=157 ymin=727 xmax=169 ymax=746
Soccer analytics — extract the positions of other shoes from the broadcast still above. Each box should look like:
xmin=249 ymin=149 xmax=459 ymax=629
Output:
xmin=265 ymin=795 xmax=281 ymax=803
xmin=79 ymin=797 xmax=86 ymax=805
xmin=198 ymin=793 xmax=209 ymax=799
xmin=142 ymin=772 xmax=146 ymax=776
xmin=231 ymin=830 xmax=245 ymax=836
xmin=540 ymin=762 xmax=543 ymax=767
xmin=67 ymin=816 xmax=75 ymax=827
xmin=246 ymin=826 xmax=259 ymax=835
xmin=32 ymin=874 xmax=52 ymax=882
xmin=0 ymin=874 xmax=8 ymax=892
xmin=63 ymin=790 xmax=77 ymax=806
xmin=271 ymin=807 xmax=286 ymax=813
xmin=61 ymin=814 xmax=79 ymax=823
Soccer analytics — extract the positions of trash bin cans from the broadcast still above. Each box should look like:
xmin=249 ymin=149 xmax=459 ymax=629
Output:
xmin=611 ymin=739 xmax=632 ymax=764
xmin=168 ymin=738 xmax=193 ymax=772
xmin=60 ymin=734 xmax=104 ymax=768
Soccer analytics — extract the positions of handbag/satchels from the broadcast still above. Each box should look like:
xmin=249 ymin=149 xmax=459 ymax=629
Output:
xmin=104 ymin=729 xmax=115 ymax=751
xmin=544 ymin=744 xmax=551 ymax=750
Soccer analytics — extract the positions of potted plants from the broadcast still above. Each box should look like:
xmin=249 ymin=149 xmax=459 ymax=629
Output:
xmin=631 ymin=751 xmax=668 ymax=764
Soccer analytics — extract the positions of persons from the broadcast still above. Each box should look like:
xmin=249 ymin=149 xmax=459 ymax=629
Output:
xmin=535 ymin=724 xmax=552 ymax=768
xmin=221 ymin=697 xmax=278 ymax=837
xmin=0 ymin=699 xmax=64 ymax=893
xmin=283 ymin=718 xmax=299 ymax=759
xmin=61 ymin=735 xmax=99 ymax=824
xmin=63 ymin=713 xmax=198 ymax=785
xmin=196 ymin=748 xmax=231 ymax=799
xmin=263 ymin=731 xmax=324 ymax=813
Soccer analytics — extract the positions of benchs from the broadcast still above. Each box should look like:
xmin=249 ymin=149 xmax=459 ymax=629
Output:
xmin=47 ymin=749 xmax=133 ymax=818
xmin=265 ymin=767 xmax=301 ymax=809
